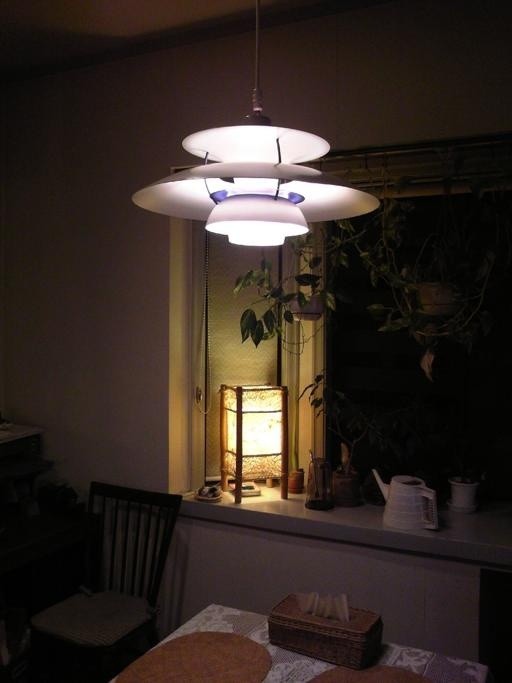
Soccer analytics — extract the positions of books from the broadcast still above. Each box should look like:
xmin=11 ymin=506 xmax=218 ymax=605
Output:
xmin=230 ymin=481 xmax=262 ymax=496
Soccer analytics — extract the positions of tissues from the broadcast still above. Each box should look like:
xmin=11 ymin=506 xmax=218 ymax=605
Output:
xmin=266 ymin=591 xmax=385 ymax=670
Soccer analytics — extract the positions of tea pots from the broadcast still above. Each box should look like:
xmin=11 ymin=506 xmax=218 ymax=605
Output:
xmin=372 ymin=469 xmax=440 ymax=532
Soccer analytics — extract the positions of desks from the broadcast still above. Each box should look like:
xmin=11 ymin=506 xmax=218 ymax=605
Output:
xmin=108 ymin=599 xmax=490 ymax=683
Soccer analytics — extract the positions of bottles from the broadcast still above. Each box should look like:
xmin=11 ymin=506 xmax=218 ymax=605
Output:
xmin=304 ymin=456 xmax=335 ymax=513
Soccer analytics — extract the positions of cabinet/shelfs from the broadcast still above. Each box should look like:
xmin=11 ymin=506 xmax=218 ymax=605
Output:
xmin=0 ymin=421 xmax=45 ymax=683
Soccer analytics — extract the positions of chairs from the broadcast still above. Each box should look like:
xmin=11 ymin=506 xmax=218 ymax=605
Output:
xmin=29 ymin=480 xmax=184 ymax=683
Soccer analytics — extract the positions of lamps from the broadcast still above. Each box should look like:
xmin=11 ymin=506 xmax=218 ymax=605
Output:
xmin=216 ymin=382 xmax=290 ymax=504
xmin=131 ymin=0 xmax=382 ymax=248
